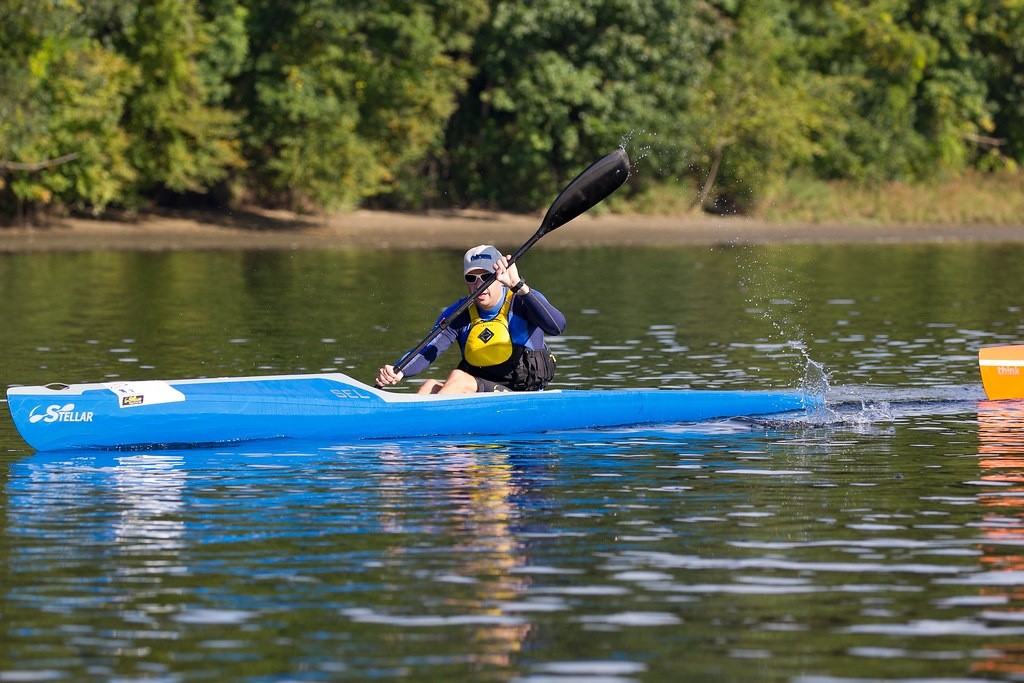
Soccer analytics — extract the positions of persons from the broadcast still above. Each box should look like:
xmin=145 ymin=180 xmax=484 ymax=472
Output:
xmin=375 ymin=244 xmax=567 ymax=394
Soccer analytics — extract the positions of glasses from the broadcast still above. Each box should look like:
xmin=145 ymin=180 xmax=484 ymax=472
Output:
xmin=464 ymin=272 xmax=492 ymax=284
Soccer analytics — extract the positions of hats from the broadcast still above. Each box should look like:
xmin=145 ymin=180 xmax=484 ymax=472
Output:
xmin=463 ymin=245 xmax=503 ymax=274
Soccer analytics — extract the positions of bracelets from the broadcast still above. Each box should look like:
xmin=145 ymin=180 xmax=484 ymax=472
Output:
xmin=510 ymin=277 xmax=525 ymax=294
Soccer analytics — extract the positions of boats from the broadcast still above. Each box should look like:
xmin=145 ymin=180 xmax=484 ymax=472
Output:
xmin=5 ymin=372 xmax=832 ymax=454
xmin=977 ymin=346 xmax=1023 ymax=403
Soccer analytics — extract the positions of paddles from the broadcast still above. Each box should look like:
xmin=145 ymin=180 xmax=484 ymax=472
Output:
xmin=376 ymin=146 xmax=630 ymax=386
